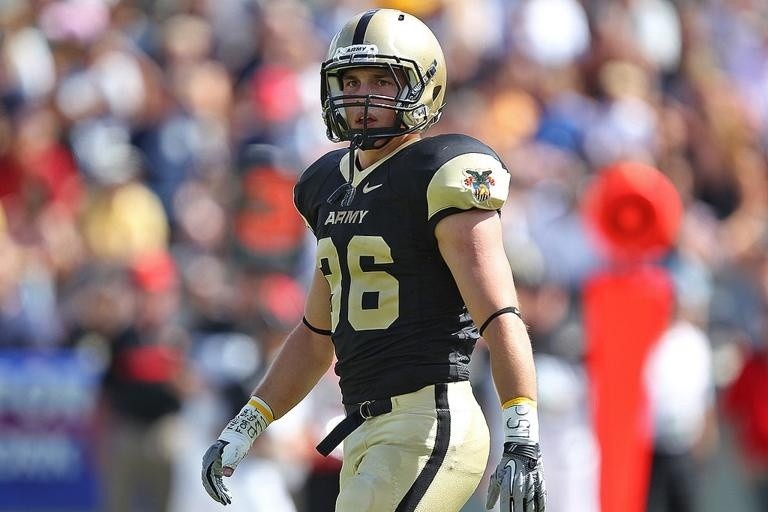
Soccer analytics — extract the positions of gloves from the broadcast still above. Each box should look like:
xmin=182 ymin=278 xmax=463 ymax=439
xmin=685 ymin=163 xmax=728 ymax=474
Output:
xmin=486 ymin=399 xmax=548 ymax=512
xmin=202 ymin=398 xmax=274 ymax=506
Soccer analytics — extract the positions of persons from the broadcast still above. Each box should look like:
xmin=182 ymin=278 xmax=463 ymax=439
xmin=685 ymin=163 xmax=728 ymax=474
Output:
xmin=0 ymin=0 xmax=767 ymax=512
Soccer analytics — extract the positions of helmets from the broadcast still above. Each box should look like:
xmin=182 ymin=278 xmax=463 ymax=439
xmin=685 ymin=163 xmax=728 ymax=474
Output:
xmin=320 ymin=9 xmax=447 ymax=151
